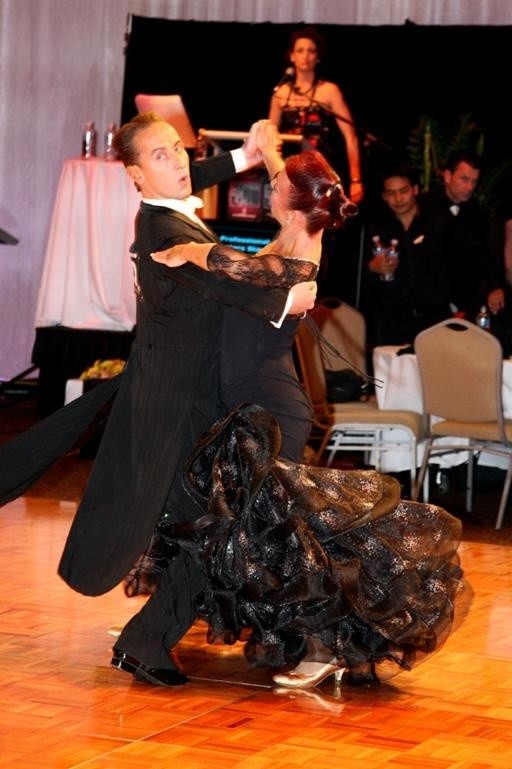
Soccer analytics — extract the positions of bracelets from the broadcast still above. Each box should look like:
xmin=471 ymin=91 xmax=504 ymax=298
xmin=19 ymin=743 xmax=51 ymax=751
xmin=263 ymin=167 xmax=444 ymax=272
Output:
xmin=349 ymin=179 xmax=361 ymax=184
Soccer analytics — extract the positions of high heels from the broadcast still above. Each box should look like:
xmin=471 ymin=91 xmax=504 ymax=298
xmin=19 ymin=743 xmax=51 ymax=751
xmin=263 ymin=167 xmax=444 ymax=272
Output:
xmin=272 ymin=654 xmax=347 ymax=691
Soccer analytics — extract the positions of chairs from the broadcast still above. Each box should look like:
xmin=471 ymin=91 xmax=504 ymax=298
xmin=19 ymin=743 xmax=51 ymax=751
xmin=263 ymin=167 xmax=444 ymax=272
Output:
xmin=294 ymin=316 xmax=431 ymax=504
xmin=413 ymin=317 xmax=512 ymax=531
xmin=310 ymin=296 xmax=370 ymax=400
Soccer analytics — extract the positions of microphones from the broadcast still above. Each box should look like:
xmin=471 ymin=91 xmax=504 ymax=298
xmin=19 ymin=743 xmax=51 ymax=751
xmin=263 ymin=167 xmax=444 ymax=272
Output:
xmin=272 ymin=67 xmax=295 ymax=93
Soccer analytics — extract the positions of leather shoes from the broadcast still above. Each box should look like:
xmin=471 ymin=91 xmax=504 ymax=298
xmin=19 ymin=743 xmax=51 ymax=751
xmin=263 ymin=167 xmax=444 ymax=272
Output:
xmin=112 ymin=649 xmax=189 ymax=687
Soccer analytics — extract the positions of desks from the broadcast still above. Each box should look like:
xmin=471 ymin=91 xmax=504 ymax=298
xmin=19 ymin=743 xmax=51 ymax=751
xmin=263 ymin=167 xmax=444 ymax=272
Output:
xmin=363 ymin=341 xmax=512 ymax=473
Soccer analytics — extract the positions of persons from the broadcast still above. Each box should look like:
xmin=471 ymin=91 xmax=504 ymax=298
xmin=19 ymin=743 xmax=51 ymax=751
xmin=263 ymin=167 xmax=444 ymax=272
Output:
xmin=267 ymin=34 xmax=367 ymax=299
xmin=56 ymin=110 xmax=317 ymax=690
xmin=362 ymin=167 xmax=461 ymax=392
xmin=126 ymin=120 xmax=474 ymax=693
xmin=425 ymin=154 xmax=510 ymax=360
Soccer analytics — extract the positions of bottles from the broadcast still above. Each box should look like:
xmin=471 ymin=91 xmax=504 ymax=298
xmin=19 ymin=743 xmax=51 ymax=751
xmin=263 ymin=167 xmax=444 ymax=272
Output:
xmin=370 ymin=235 xmax=400 ymax=280
xmin=477 ymin=305 xmax=490 ymax=330
xmin=102 ymin=124 xmax=117 ymax=161
xmin=82 ymin=121 xmax=97 ymax=160
